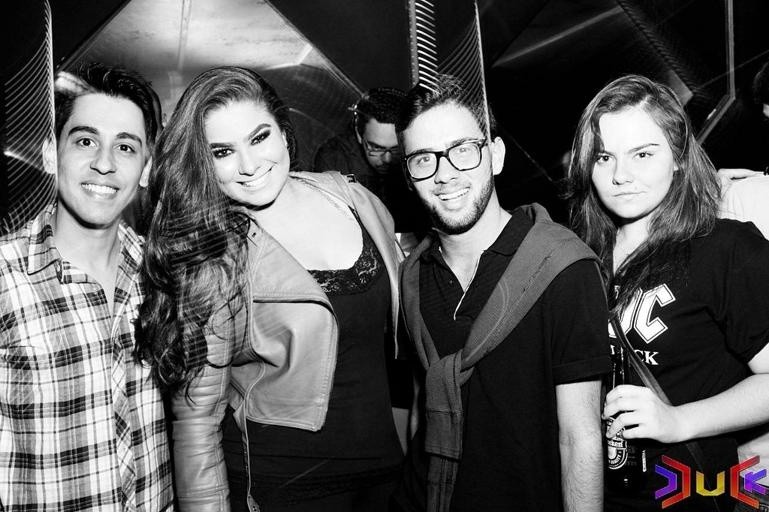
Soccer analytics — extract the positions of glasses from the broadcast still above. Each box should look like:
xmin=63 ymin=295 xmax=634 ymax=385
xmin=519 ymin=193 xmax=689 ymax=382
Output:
xmin=403 ymin=139 xmax=486 ymax=181
xmin=363 ymin=137 xmax=399 ymax=156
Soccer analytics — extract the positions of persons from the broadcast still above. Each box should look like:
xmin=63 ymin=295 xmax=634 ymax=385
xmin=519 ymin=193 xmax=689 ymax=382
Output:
xmin=398 ymin=74 xmax=613 ymax=511
xmin=0 ymin=63 xmax=175 ymax=512
xmin=313 ymin=87 xmax=407 ymax=210
xmin=132 ymin=64 xmax=404 ymax=512
xmin=554 ymin=72 xmax=769 ymax=512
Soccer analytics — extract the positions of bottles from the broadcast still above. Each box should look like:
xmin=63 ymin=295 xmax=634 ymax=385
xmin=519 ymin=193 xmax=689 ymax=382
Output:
xmin=600 ymin=353 xmax=645 ymax=491
